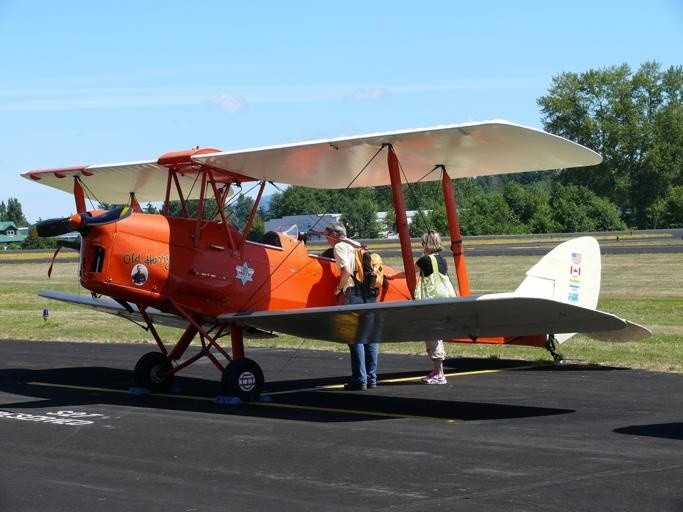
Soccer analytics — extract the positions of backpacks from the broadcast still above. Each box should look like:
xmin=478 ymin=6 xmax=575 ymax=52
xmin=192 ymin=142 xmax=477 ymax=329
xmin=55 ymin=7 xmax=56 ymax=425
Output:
xmin=334 ymin=240 xmax=383 ymax=298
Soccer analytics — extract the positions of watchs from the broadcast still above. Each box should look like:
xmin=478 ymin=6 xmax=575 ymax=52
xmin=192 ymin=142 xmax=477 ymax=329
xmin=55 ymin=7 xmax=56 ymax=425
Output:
xmin=336 ymin=285 xmax=342 ymax=291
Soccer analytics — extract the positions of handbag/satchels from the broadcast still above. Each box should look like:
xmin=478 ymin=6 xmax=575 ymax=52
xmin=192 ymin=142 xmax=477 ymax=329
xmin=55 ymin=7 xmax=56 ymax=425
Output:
xmin=413 ymin=256 xmax=456 ymax=300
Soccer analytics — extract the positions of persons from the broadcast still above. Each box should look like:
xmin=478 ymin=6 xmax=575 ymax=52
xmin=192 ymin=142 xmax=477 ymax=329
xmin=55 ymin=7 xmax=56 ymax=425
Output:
xmin=382 ymin=230 xmax=449 ymax=385
xmin=321 ymin=221 xmax=378 ymax=391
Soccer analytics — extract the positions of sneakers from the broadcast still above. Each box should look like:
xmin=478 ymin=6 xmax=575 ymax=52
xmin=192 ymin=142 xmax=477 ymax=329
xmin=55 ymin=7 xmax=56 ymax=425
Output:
xmin=421 ymin=371 xmax=447 ymax=385
xmin=344 ymin=384 xmax=377 ymax=391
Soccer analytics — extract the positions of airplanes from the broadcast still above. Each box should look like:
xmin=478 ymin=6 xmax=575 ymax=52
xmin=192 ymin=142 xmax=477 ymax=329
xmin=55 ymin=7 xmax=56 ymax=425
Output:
xmin=15 ymin=118 xmax=651 ymax=401
